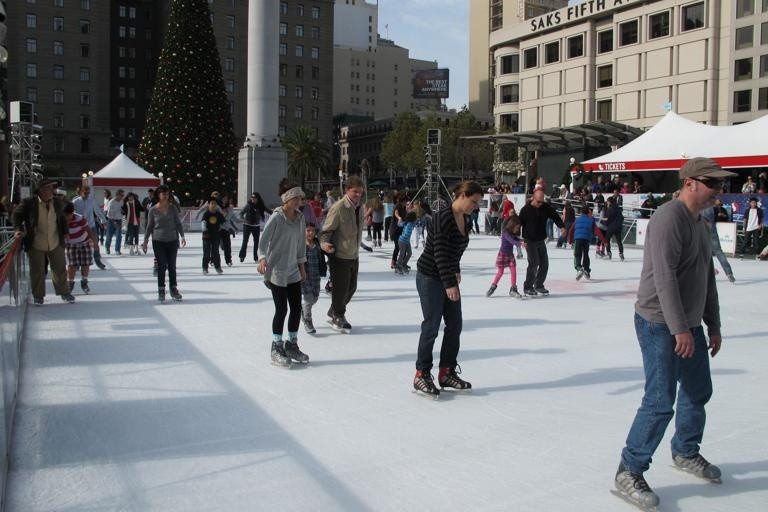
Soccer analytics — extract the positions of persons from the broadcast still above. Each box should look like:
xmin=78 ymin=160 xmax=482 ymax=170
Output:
xmin=467 ymin=172 xmax=657 ymax=299
xmin=613 ymin=155 xmax=724 ymax=510
xmin=713 ymin=172 xmax=767 ymax=262
xmin=295 ymin=190 xmax=338 ymax=335
xmin=363 ymin=187 xmax=448 ymax=274
xmin=193 ymin=190 xmax=274 ymax=275
xmin=411 ymin=181 xmax=485 ymax=396
xmin=11 ymin=179 xmax=185 ymax=307
xmin=257 ymin=177 xmax=311 ymax=366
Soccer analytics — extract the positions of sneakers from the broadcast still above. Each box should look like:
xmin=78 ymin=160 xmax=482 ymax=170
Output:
xmin=204 ymin=261 xmax=232 ymax=274
xmin=106 ymin=247 xmax=139 ymax=255
xmin=95 ymin=260 xmax=105 ymax=268
xmin=159 ymin=287 xmax=165 ymax=300
xmin=34 ymin=280 xmax=89 ymax=304
xmin=414 ymin=368 xmax=440 ymax=395
xmin=438 ymin=367 xmax=471 ymax=390
xmin=486 ymin=284 xmax=549 ymax=298
xmin=614 ymin=462 xmax=659 ymax=507
xmin=271 ymin=341 xmax=309 ymax=365
xmin=391 ymin=259 xmax=411 ymax=275
xmin=576 ymin=267 xmax=590 ymax=280
xmin=332 ymin=316 xmax=351 ymax=329
xmin=595 ymin=250 xmax=624 ymax=261
xmin=304 ymin=313 xmax=315 ymax=333
xmin=672 ymin=451 xmax=721 ymax=479
xmin=170 ymin=287 xmax=182 ymax=299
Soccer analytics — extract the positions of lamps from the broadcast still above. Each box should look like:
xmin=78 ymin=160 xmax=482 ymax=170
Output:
xmin=423 ymin=146 xmax=431 ymax=180
xmin=30 ymin=124 xmax=45 ymax=182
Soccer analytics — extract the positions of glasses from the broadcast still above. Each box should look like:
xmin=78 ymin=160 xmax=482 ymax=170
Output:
xmin=690 ymin=177 xmax=725 ymax=189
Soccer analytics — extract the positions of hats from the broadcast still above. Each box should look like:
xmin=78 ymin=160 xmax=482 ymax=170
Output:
xmin=679 ymin=157 xmax=738 ymax=180
xmin=279 ymin=178 xmax=305 ymax=203
xmin=32 ymin=179 xmax=58 ymax=193
xmin=503 ymin=199 xmax=514 ymax=215
